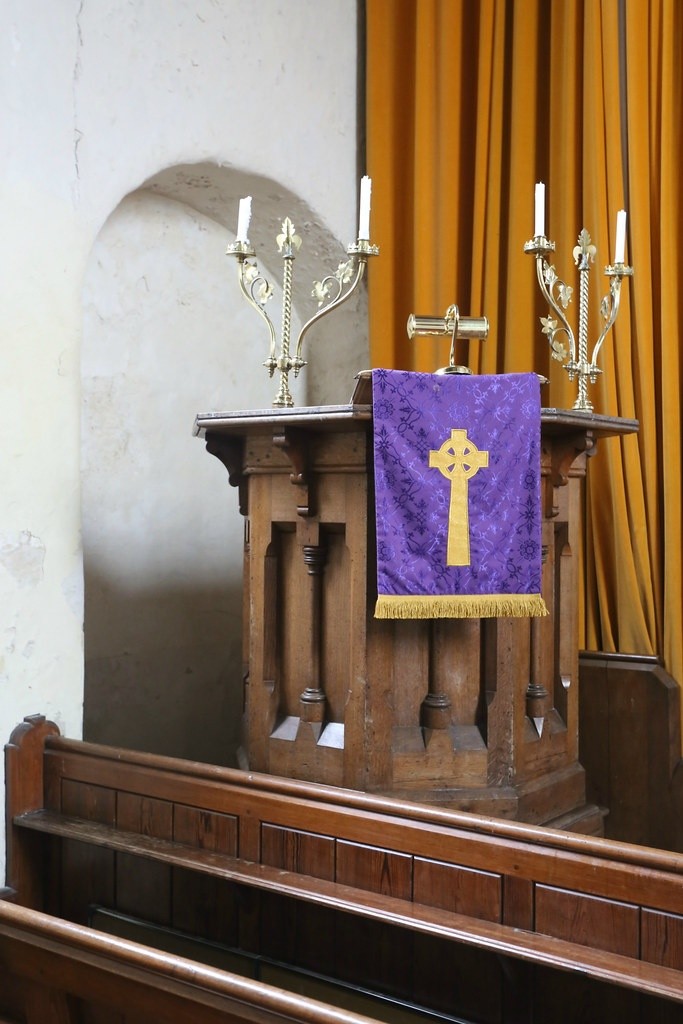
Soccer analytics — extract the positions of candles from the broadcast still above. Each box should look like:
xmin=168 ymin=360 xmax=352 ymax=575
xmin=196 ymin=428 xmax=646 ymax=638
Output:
xmin=236 ymin=196 xmax=251 ymax=240
xmin=534 ymin=182 xmax=546 ymax=235
xmin=359 ymin=174 xmax=372 ymax=240
xmin=615 ymin=209 xmax=627 ymax=264
